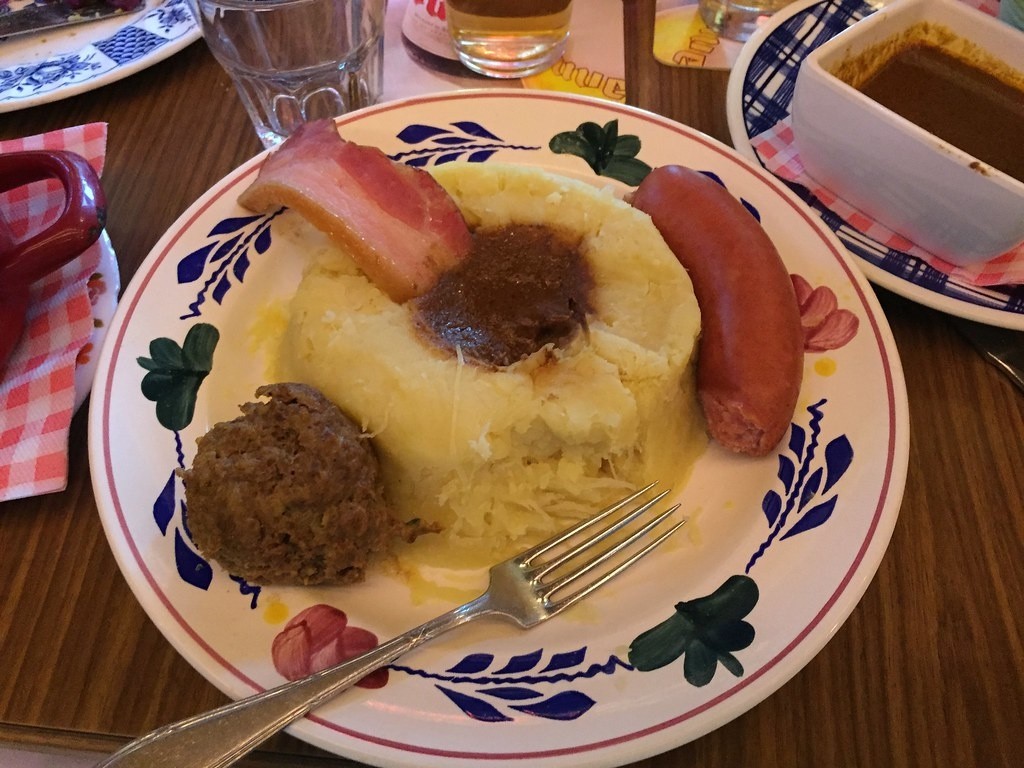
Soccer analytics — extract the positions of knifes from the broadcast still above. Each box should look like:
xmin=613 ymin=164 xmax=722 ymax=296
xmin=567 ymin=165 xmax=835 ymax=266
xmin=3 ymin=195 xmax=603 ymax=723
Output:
xmin=0 ymin=0 xmax=146 ymax=37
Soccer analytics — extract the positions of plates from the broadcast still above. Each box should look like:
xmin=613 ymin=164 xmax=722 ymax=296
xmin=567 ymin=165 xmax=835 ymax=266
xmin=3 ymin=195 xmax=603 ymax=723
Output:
xmin=727 ymin=0 xmax=1024 ymax=332
xmin=0 ymin=0 xmax=202 ymax=113
xmin=88 ymin=88 xmax=911 ymax=768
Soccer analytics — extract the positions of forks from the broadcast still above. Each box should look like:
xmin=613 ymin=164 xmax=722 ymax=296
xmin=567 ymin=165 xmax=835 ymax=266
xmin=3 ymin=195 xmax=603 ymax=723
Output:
xmin=94 ymin=480 xmax=686 ymax=768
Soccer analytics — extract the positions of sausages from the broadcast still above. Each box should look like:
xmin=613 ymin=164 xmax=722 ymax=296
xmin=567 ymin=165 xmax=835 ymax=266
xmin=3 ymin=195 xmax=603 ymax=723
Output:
xmin=632 ymin=164 xmax=804 ymax=457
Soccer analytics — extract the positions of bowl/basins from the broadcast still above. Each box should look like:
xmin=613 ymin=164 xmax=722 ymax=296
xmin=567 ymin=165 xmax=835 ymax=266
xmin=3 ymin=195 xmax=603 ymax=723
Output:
xmin=793 ymin=0 xmax=1024 ymax=268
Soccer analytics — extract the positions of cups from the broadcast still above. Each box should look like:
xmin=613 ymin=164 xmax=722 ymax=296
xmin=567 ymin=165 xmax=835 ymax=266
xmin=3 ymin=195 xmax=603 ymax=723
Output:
xmin=699 ymin=0 xmax=796 ymax=42
xmin=444 ymin=0 xmax=574 ymax=79
xmin=187 ymin=1 xmax=387 ymax=150
